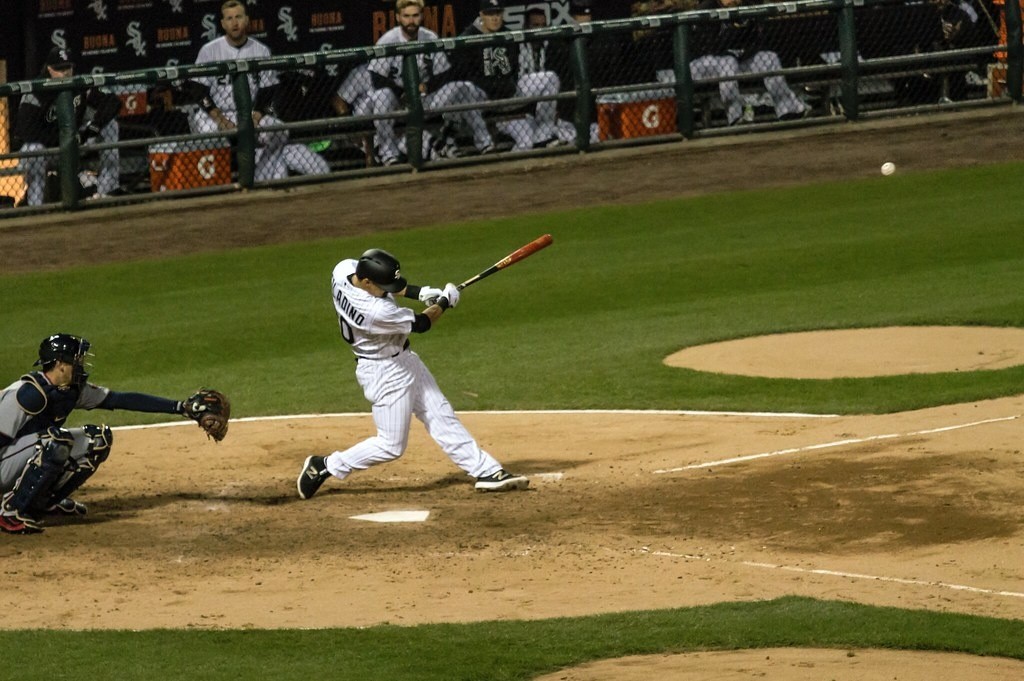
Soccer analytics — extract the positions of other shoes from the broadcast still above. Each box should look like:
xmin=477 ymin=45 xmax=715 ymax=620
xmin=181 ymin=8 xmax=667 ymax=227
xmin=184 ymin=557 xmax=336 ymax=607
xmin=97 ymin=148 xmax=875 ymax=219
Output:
xmin=730 ymin=115 xmax=750 ymax=127
xmin=479 ymin=145 xmax=495 ymax=156
xmin=384 ymin=157 xmax=399 ymax=167
xmin=779 ymin=102 xmax=823 ymax=122
xmin=83 ymin=192 xmax=113 ymax=201
xmin=533 ymin=137 xmax=559 ymax=149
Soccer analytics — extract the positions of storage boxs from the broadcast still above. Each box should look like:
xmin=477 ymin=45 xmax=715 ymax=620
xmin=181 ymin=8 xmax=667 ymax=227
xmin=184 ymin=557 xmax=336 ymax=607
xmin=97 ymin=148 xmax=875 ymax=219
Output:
xmin=148 ymin=138 xmax=232 ymax=193
xmin=595 ymin=88 xmax=677 ymax=142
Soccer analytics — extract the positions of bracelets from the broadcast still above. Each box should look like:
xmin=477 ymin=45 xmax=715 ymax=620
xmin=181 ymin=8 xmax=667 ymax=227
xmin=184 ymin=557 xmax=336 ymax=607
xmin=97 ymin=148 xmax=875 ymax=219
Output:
xmin=404 ymin=285 xmax=421 ymax=300
xmin=437 ymin=296 xmax=449 ymax=311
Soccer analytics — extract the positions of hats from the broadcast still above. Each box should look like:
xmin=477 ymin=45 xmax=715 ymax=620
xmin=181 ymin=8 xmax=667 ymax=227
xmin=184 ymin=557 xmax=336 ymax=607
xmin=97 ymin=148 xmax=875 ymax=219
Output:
xmin=481 ymin=1 xmax=503 ymax=15
xmin=46 ymin=47 xmax=74 ymax=70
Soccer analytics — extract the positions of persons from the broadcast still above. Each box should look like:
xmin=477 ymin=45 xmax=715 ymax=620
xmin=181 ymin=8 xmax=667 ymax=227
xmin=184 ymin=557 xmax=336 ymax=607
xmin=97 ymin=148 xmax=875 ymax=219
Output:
xmin=17 ymin=0 xmax=1000 ymax=208
xmin=297 ymin=248 xmax=529 ymax=501
xmin=0 ymin=334 xmax=230 ymax=534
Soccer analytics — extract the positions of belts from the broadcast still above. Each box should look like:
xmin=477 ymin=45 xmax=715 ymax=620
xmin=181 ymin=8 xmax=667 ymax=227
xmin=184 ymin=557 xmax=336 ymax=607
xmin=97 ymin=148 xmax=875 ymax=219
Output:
xmin=359 ymin=338 xmax=411 ymax=359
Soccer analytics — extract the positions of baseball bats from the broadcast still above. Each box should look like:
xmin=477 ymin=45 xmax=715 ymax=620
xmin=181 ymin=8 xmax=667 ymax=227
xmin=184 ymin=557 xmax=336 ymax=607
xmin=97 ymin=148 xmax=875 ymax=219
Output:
xmin=429 ymin=233 xmax=554 ymax=306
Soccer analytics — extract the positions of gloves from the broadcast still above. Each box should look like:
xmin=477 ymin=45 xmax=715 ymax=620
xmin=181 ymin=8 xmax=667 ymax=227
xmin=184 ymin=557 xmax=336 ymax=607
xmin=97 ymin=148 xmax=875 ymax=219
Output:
xmin=417 ymin=285 xmax=443 ymax=309
xmin=435 ymin=282 xmax=459 ymax=308
xmin=73 ymin=125 xmax=99 ymax=146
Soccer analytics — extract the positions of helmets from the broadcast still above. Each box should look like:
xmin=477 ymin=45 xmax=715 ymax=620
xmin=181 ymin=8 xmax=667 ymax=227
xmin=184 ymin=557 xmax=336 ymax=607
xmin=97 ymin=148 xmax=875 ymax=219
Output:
xmin=33 ymin=332 xmax=91 ymax=366
xmin=355 ymin=248 xmax=407 ymax=292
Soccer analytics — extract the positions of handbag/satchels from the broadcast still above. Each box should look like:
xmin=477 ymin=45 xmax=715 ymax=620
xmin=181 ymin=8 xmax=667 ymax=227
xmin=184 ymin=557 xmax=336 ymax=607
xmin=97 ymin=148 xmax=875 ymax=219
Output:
xmin=296 ymin=455 xmax=330 ymax=500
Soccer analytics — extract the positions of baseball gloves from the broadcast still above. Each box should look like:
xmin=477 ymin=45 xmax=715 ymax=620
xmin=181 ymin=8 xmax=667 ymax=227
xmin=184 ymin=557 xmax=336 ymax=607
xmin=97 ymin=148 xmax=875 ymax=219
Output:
xmin=199 ymin=388 xmax=231 ymax=441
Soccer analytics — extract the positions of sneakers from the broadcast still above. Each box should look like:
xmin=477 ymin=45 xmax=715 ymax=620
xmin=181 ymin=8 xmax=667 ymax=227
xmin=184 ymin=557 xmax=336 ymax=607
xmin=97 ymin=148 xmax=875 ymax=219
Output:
xmin=36 ymin=498 xmax=88 ymax=523
xmin=475 ymin=468 xmax=529 ymax=493
xmin=0 ymin=506 xmax=41 ymax=535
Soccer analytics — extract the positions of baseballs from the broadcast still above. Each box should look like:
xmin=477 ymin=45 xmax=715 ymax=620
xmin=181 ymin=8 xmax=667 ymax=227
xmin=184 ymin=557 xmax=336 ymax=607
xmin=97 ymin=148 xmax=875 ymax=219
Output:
xmin=880 ymin=162 xmax=896 ymax=176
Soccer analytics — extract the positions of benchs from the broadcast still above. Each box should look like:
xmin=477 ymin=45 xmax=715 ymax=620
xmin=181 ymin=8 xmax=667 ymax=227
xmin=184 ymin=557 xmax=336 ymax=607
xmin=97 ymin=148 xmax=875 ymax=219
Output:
xmin=0 ymin=56 xmax=979 ymax=182
